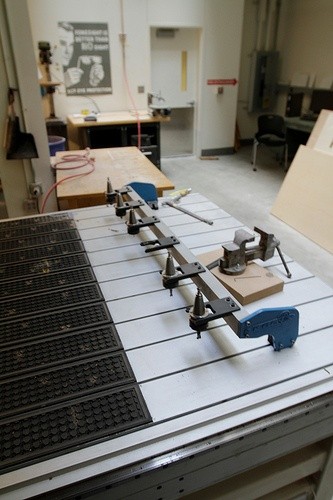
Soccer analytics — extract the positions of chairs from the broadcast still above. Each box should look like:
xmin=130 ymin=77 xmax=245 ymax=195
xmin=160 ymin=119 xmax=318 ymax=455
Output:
xmin=251 ymin=114 xmax=289 ymax=172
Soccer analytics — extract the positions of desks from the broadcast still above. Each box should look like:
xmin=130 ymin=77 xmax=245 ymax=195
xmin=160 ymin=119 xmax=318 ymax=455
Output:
xmin=150 ymin=97 xmax=195 ymax=109
xmin=56 ymin=145 xmax=176 ymax=211
xmin=67 ymin=108 xmax=171 ymax=149
xmin=284 ymin=113 xmax=316 ymax=139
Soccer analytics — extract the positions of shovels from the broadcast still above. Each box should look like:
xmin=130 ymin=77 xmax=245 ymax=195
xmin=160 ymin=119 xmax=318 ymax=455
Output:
xmin=5 ymin=115 xmax=40 ymax=161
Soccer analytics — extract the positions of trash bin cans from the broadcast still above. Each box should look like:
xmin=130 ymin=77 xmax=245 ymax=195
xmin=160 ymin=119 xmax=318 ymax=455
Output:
xmin=48 ymin=136 xmax=66 ymax=156
